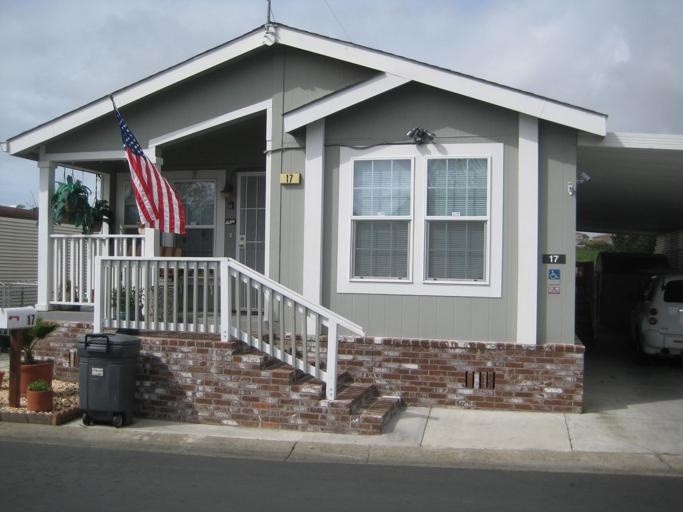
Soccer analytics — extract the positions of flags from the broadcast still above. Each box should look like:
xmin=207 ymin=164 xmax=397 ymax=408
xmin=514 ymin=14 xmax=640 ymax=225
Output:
xmin=112 ymin=98 xmax=185 ymax=235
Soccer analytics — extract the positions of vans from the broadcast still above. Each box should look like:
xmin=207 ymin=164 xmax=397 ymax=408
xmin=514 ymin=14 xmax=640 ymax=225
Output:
xmin=574 ymin=252 xmax=669 ymax=352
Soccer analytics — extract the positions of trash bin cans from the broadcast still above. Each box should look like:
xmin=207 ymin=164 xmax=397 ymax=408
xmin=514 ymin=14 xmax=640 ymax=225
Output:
xmin=77 ymin=333 xmax=141 ymax=427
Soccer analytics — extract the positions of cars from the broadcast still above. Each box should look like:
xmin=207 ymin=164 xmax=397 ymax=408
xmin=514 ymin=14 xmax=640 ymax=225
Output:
xmin=630 ymin=273 xmax=683 ymax=362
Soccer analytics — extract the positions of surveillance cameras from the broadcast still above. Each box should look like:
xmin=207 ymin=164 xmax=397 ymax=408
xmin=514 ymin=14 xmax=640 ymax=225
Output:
xmin=581 ymin=172 xmax=592 ymax=184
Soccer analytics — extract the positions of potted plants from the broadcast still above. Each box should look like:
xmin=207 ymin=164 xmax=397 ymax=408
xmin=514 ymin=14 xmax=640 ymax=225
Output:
xmin=18 ymin=315 xmax=63 ymax=412
xmin=59 ymin=279 xmax=78 ymax=310
xmin=50 ymin=175 xmax=112 ymax=234
xmin=111 ymin=286 xmax=144 ymax=321
